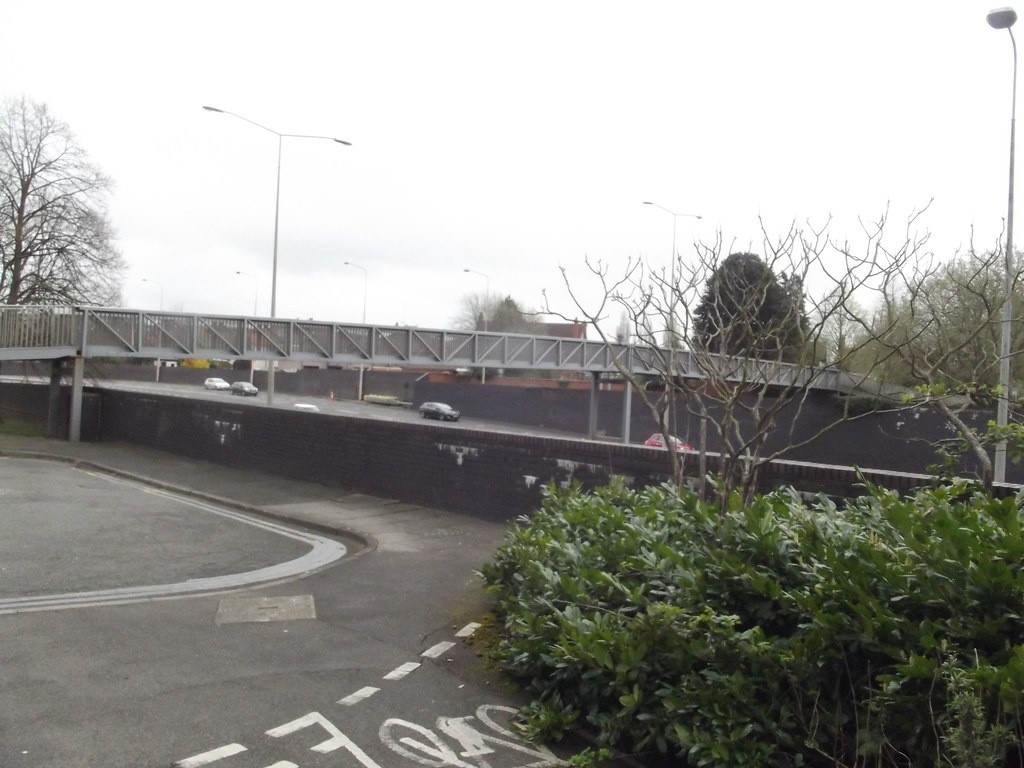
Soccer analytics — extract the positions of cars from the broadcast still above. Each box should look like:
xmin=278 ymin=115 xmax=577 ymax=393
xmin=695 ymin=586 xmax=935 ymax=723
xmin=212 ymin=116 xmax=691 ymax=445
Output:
xmin=204 ymin=377 xmax=230 ymax=391
xmin=419 ymin=402 xmax=461 ymax=422
xmin=231 ymin=382 xmax=259 ymax=397
xmin=644 ymin=433 xmax=694 ymax=452
xmin=294 ymin=404 xmax=321 ymax=413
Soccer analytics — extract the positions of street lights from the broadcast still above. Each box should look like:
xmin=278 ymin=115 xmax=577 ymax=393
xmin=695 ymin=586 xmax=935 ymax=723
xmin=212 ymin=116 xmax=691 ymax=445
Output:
xmin=203 ymin=104 xmax=352 ymax=405
xmin=986 ymin=6 xmax=1019 ymax=483
xmin=641 ymin=201 xmax=703 ymax=434
